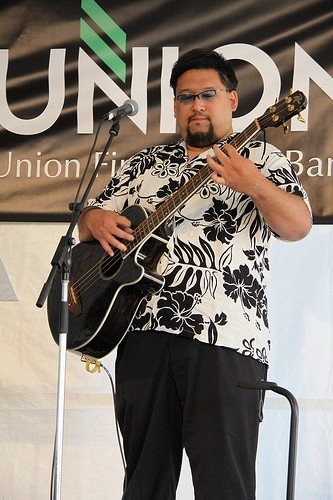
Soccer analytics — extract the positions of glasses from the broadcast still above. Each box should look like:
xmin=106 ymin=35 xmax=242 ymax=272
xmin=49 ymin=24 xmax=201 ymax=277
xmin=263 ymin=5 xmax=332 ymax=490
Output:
xmin=174 ymin=86 xmax=230 ymax=105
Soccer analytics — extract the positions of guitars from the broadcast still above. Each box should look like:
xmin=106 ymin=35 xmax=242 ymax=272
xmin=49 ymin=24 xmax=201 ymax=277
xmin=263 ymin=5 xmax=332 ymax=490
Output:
xmin=47 ymin=90 xmax=308 ymax=360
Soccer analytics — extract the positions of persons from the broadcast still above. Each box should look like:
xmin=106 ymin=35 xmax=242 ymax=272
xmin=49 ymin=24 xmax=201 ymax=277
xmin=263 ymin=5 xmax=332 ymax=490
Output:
xmin=77 ymin=49 xmax=314 ymax=500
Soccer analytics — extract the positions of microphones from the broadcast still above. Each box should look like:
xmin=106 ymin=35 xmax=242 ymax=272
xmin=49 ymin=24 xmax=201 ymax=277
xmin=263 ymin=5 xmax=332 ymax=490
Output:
xmin=102 ymin=99 xmax=139 ymax=120
